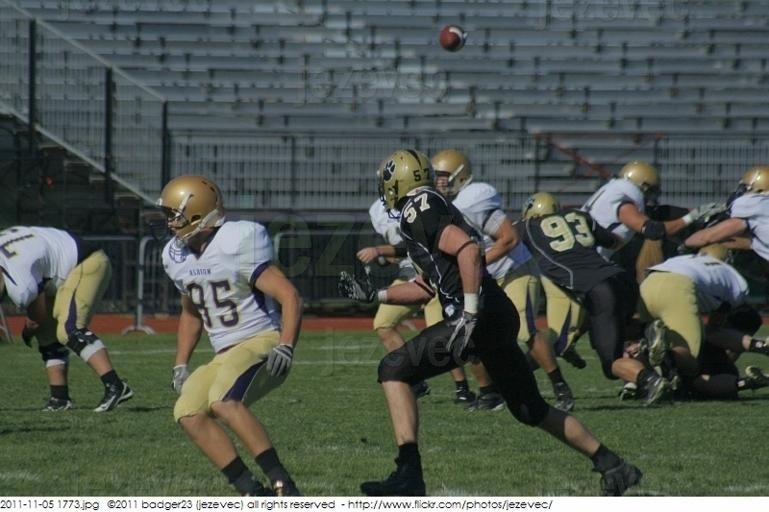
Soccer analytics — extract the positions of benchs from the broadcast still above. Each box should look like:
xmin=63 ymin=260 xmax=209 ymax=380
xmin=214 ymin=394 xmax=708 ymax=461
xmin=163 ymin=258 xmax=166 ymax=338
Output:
xmin=1 ymin=0 xmax=768 ymax=227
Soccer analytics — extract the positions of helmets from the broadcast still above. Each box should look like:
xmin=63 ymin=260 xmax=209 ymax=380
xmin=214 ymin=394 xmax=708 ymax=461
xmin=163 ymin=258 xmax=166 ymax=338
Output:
xmin=155 ymin=176 xmax=227 ymax=248
xmin=734 ymin=164 xmax=769 ymax=198
xmin=619 ymin=160 xmax=662 ymax=210
xmin=376 ymin=149 xmax=473 ymax=219
xmin=522 ymin=191 xmax=559 ymax=221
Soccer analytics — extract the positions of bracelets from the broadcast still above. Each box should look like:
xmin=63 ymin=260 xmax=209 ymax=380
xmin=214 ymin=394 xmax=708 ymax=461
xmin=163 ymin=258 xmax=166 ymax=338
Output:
xmin=375 ymin=247 xmax=381 ymax=256
xmin=463 ymin=292 xmax=482 ymax=314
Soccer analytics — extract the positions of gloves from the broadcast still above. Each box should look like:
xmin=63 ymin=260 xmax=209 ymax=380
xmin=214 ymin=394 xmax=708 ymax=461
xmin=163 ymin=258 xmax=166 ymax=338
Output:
xmin=338 ymin=265 xmax=378 ymax=304
xmin=171 ymin=365 xmax=189 ymax=394
xmin=22 ymin=322 xmax=36 ymax=348
xmin=690 ymin=201 xmax=727 ymax=223
xmin=258 ymin=342 xmax=294 ymax=379
xmin=445 ymin=310 xmax=486 ymax=365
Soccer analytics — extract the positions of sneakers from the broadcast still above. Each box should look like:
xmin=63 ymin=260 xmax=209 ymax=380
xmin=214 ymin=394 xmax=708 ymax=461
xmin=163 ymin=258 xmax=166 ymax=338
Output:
xmin=360 ymin=471 xmax=425 ymax=497
xmin=562 ymin=347 xmax=586 ymax=370
xmin=94 ymin=380 xmax=134 ymax=413
xmin=454 ymin=389 xmax=505 ymax=412
xmin=413 ymin=380 xmax=431 ymax=397
xmin=43 ymin=397 xmax=72 ymax=412
xmin=618 ymin=318 xmax=692 ymax=406
xmin=599 ymin=462 xmax=642 ymax=496
xmin=240 ymin=480 xmax=299 ymax=496
xmin=744 ymin=364 xmax=768 ymax=389
xmin=554 ymin=392 xmax=574 ymax=411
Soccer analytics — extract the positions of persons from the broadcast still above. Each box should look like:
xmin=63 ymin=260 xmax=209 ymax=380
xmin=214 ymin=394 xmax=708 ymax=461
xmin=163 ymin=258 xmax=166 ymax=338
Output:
xmin=335 ymin=147 xmax=647 ymax=496
xmin=155 ymin=173 xmax=307 ymax=495
xmin=0 ymin=225 xmax=135 ymax=415
xmin=355 ymin=148 xmax=768 ymax=417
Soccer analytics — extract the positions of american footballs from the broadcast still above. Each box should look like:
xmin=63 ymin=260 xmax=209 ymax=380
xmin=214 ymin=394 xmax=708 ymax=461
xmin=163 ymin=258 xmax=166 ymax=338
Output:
xmin=439 ymin=26 xmax=466 ymax=51
